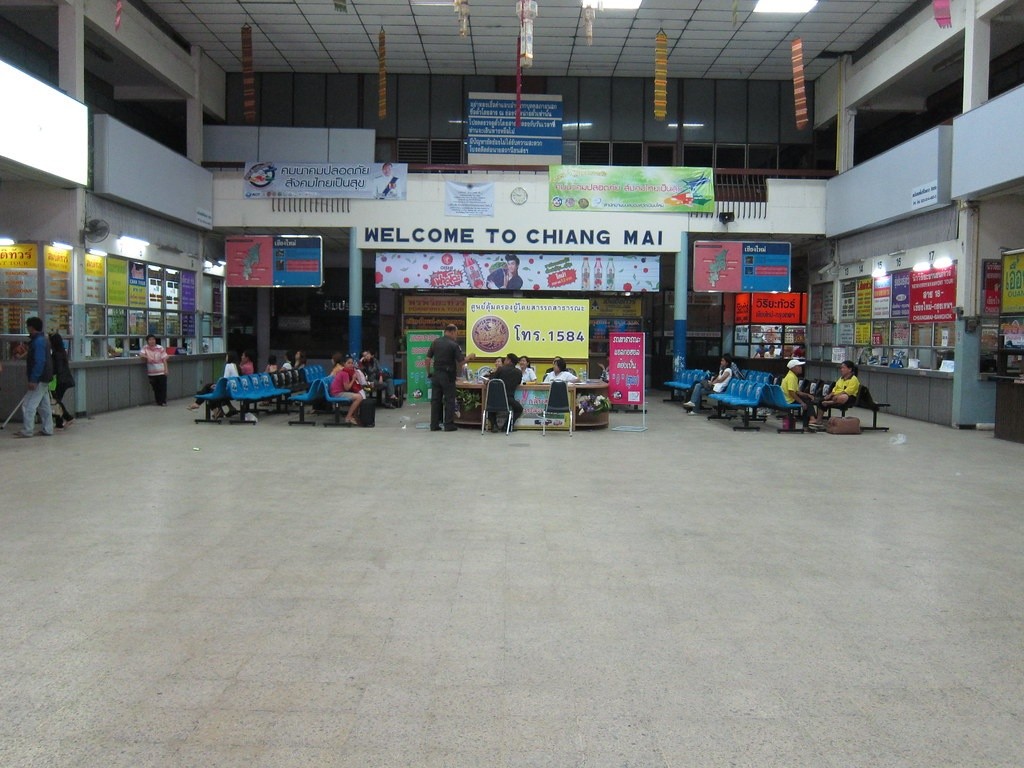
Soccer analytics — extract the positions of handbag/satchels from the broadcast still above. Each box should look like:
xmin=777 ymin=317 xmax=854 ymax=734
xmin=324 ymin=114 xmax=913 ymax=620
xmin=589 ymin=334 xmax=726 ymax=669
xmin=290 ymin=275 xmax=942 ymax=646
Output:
xmin=826 ymin=416 xmax=860 ymax=434
xmin=700 ymin=378 xmax=714 ymax=391
xmin=32 ymin=385 xmax=64 ymax=418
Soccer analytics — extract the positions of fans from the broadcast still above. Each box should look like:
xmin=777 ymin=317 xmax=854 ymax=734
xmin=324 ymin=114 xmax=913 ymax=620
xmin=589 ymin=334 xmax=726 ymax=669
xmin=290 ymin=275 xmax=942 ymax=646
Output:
xmin=79 ymin=219 xmax=110 ymax=244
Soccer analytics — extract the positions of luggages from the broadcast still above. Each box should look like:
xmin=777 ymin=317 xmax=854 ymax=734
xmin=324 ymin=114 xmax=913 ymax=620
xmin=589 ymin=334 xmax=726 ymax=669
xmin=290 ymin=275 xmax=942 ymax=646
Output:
xmin=359 ymin=395 xmax=376 ymax=427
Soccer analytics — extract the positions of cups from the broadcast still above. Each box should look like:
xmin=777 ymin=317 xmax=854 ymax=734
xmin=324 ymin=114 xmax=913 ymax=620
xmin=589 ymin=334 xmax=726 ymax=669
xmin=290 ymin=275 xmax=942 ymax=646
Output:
xmin=468 ymin=369 xmax=472 ymax=381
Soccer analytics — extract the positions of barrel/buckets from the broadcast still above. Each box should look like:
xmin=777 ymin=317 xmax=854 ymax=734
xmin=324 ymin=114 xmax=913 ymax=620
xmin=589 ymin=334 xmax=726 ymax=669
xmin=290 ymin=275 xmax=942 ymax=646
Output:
xmin=166 ymin=347 xmax=176 ymax=355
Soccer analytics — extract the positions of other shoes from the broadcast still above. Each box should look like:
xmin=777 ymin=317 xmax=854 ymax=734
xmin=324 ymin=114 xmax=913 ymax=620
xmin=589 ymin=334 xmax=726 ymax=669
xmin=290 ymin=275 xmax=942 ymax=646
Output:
xmin=345 ymin=416 xmax=359 ymax=425
xmin=502 ymin=422 xmax=508 ymax=432
xmin=54 ymin=414 xmax=75 ymax=431
xmin=682 ymin=400 xmax=696 ymax=408
xmin=490 ymin=423 xmax=499 ymax=432
xmin=444 ymin=427 xmax=458 ymax=431
xmin=33 ymin=430 xmax=51 ymax=437
xmin=10 ymin=431 xmax=30 ymax=438
xmin=186 ymin=405 xmax=200 ymax=412
xmin=801 ymin=428 xmax=817 ymax=434
xmin=430 ymin=427 xmax=442 ymax=431
xmin=687 ymin=410 xmax=699 ymax=416
xmin=808 ymin=421 xmax=824 ymax=431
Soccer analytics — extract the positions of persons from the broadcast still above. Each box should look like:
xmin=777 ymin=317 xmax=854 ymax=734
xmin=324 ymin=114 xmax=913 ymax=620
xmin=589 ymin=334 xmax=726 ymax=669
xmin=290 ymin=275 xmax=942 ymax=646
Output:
xmin=780 ymin=360 xmax=822 ymax=433
xmin=764 ymin=345 xmax=777 ymax=358
xmin=486 ymin=353 xmax=537 ymax=433
xmin=372 ymin=163 xmax=401 ymax=199
xmin=808 ymin=361 xmax=860 ymax=424
xmin=210 ymin=350 xmax=254 ymax=416
xmin=37 ymin=331 xmax=75 ymax=430
xmin=487 ymin=252 xmax=523 ymax=289
xmin=425 ymin=323 xmax=475 ymax=431
xmin=791 ymin=344 xmax=806 ymax=359
xmin=139 ymin=334 xmax=169 ymax=406
xmin=980 ymin=354 xmax=997 ymax=373
xmin=598 ymin=351 xmax=609 ymax=382
xmin=542 ymin=356 xmax=580 ymax=383
xmin=724 ymin=353 xmax=746 ymax=380
xmin=306 ymin=348 xmax=398 ymax=425
xmin=294 ymin=349 xmax=306 ymax=380
xmin=278 ymin=351 xmax=295 ymax=385
xmin=91 ymin=331 xmax=121 ymax=358
xmin=683 ymin=356 xmax=732 ymax=416
xmin=14 ymin=317 xmax=54 ymax=438
xmin=186 ymin=351 xmax=239 ymax=420
xmin=756 ymin=359 xmax=789 ymax=416
xmin=259 ymin=355 xmax=281 ymax=406
xmin=753 ymin=342 xmax=769 ymax=358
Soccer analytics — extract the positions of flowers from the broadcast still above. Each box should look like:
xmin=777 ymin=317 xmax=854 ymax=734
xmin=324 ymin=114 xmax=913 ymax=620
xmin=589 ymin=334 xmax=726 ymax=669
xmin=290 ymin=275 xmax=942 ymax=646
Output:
xmin=577 ymin=389 xmax=610 ymax=414
xmin=453 ymin=389 xmax=481 ymax=417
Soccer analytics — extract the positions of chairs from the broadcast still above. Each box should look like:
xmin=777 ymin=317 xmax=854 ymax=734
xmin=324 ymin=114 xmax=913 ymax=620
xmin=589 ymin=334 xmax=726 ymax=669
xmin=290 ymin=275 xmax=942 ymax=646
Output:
xmin=663 ymin=368 xmax=891 ymax=434
xmin=193 ymin=363 xmax=407 ymax=427
xmin=477 ymin=366 xmax=579 ymax=436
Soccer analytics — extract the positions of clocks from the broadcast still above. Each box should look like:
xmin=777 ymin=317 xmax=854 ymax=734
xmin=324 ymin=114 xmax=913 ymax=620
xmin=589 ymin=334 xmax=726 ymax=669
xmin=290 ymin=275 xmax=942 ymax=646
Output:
xmin=511 ymin=187 xmax=528 ymax=205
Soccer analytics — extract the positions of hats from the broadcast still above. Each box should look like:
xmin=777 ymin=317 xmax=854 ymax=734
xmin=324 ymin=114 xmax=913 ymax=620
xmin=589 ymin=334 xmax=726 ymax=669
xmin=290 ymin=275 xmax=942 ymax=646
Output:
xmin=786 ymin=359 xmax=806 ymax=369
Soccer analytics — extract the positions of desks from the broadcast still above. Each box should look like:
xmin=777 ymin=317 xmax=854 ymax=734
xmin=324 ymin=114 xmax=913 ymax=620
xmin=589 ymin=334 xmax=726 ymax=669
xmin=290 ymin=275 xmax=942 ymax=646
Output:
xmin=452 ymin=377 xmax=609 ymax=429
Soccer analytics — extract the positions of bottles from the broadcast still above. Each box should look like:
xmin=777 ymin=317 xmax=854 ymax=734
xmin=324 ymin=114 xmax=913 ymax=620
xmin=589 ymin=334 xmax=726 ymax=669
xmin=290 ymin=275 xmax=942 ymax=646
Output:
xmin=463 ymin=253 xmax=484 ymax=289
xmin=583 ymin=372 xmax=587 ymax=381
xmin=474 ymin=369 xmax=479 ymax=383
xmin=606 ymin=258 xmax=615 ymax=290
xmin=581 ymin=257 xmax=590 ymax=290
xmin=463 ymin=363 xmax=468 ymax=379
xmin=594 ymin=258 xmax=602 ymax=290
xmin=579 ymin=368 xmax=583 ymax=382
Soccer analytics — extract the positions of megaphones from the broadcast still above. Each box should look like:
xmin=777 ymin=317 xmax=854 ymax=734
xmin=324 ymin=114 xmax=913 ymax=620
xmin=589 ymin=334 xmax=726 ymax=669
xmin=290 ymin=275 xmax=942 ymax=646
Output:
xmin=719 ymin=212 xmax=734 ymax=224
